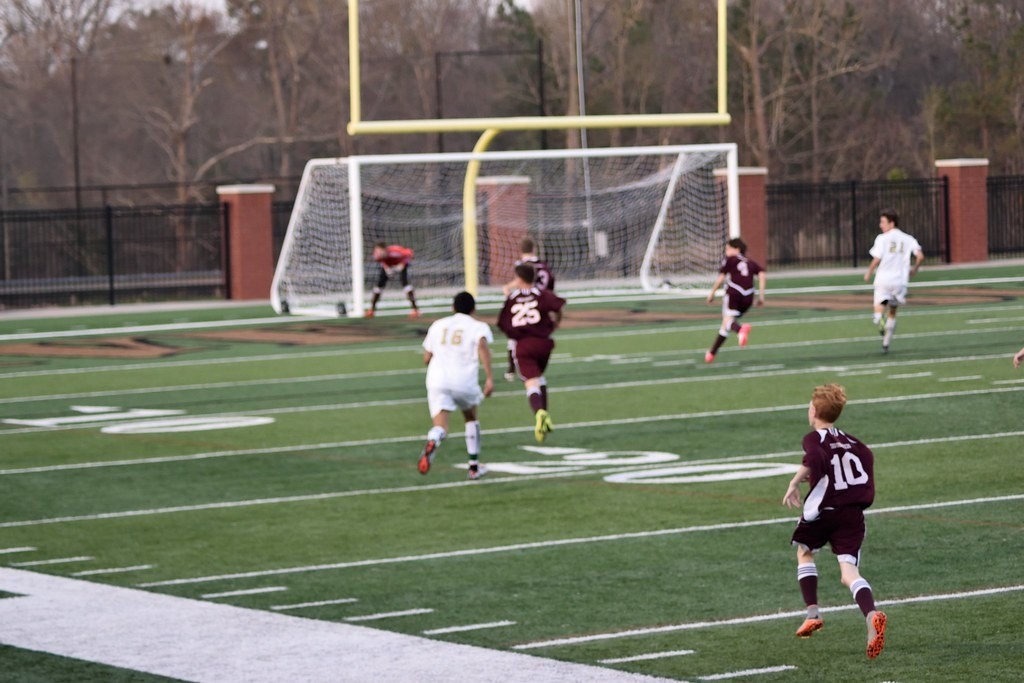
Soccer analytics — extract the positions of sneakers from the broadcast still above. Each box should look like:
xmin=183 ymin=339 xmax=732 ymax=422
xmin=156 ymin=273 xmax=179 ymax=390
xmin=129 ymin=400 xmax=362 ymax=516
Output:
xmin=534 ymin=409 xmax=552 ymax=443
xmin=795 ymin=615 xmax=824 ymax=638
xmin=866 ymin=609 xmax=886 ymax=658
xmin=469 ymin=465 xmax=488 ymax=478
xmin=417 ymin=440 xmax=438 ymax=475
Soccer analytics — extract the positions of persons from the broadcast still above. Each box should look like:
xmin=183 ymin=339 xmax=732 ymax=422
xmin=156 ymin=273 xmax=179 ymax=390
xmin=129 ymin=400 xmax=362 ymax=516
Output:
xmin=364 ymin=243 xmax=421 ymax=318
xmin=783 ymin=384 xmax=887 ymax=659
xmin=496 ymin=265 xmax=563 ymax=444
xmin=1013 ymin=348 xmax=1024 ymax=369
xmin=417 ymin=291 xmax=495 ymax=480
xmin=863 ymin=211 xmax=925 ymax=355
xmin=704 ymin=238 xmax=766 ymax=364
xmin=503 ymin=240 xmax=555 ymax=384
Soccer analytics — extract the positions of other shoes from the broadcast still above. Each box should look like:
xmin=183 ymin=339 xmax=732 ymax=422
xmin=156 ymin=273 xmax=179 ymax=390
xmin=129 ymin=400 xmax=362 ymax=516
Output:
xmin=364 ymin=309 xmax=374 ymax=317
xmin=878 ymin=314 xmax=887 ymax=335
xmin=408 ymin=309 xmax=419 ymax=317
xmin=504 ymin=371 xmax=513 ymax=381
xmin=738 ymin=323 xmax=751 ymax=347
xmin=705 ymin=350 xmax=713 ymax=363
xmin=881 ymin=344 xmax=889 ymax=354
